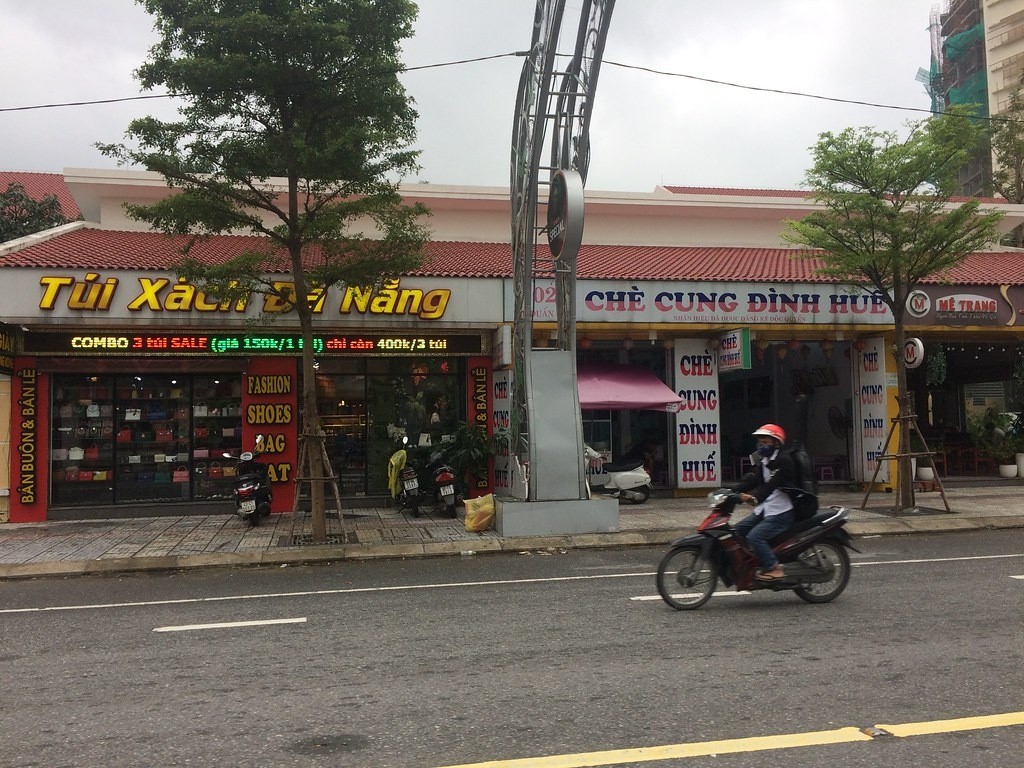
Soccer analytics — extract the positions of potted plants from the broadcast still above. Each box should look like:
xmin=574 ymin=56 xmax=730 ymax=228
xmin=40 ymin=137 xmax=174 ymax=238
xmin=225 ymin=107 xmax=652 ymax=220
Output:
xmin=1008 ymin=429 xmax=1024 ymax=477
xmin=990 ymin=440 xmax=1017 ymax=478
xmin=917 ymin=447 xmax=937 ymax=481
xmin=909 ymin=437 xmax=924 ymax=481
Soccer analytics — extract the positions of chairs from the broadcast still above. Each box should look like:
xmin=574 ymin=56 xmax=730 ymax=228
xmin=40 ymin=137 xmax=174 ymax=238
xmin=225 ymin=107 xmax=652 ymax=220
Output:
xmin=917 ymin=448 xmax=947 ymax=477
xmin=957 ymin=447 xmax=992 ymax=477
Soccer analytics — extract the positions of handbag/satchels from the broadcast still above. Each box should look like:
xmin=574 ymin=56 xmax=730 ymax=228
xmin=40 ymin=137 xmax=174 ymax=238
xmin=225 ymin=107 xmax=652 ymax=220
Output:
xmin=779 ymin=487 xmax=819 ymax=522
xmin=51 ymin=374 xmax=243 ymax=486
xmin=418 ymin=433 xmax=432 ymax=447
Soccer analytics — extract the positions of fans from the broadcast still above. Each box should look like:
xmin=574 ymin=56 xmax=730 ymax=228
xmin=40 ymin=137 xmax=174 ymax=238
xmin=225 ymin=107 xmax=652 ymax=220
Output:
xmin=828 ymin=405 xmax=855 ymax=481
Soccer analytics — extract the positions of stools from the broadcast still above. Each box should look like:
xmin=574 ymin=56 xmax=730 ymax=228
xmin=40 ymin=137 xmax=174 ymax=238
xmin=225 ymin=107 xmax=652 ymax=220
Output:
xmin=816 ymin=466 xmax=836 ymax=481
xmin=722 ymin=466 xmax=733 ymax=480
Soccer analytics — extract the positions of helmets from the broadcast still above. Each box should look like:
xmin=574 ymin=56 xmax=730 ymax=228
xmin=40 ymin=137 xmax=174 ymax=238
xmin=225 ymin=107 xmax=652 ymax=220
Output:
xmin=752 ymin=423 xmax=786 ymax=446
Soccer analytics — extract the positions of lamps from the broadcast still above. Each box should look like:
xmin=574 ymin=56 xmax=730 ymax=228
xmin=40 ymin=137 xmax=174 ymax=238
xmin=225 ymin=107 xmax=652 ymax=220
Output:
xmin=648 ymin=332 xmax=658 ymax=346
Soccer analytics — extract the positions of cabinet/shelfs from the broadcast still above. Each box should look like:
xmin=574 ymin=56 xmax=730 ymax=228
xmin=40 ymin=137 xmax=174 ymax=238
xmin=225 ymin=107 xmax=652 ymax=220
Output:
xmin=53 ymin=372 xmax=370 ymax=504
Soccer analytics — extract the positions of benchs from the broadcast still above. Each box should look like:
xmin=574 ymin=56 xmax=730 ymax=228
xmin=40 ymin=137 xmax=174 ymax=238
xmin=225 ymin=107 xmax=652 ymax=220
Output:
xmin=652 ymin=468 xmax=669 ymax=487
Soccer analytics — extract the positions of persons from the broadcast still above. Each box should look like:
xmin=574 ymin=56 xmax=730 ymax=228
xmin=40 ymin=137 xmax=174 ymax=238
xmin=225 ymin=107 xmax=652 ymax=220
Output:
xmin=731 ymin=424 xmax=795 ymax=581
xmin=986 ymin=422 xmax=1005 ymax=444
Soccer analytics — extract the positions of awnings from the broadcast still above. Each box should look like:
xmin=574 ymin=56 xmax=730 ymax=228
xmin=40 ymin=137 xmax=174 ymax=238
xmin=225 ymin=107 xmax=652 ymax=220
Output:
xmin=577 ymin=360 xmax=683 ymax=413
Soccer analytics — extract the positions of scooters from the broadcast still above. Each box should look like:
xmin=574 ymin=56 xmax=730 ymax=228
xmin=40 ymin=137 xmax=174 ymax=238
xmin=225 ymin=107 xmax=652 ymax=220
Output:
xmin=583 ymin=443 xmax=654 ymax=503
xmin=223 ymin=434 xmax=272 ymax=527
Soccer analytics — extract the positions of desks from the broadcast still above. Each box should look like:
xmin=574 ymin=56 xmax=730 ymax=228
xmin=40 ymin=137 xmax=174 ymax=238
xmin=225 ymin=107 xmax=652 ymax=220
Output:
xmin=732 ymin=457 xmax=752 ymax=481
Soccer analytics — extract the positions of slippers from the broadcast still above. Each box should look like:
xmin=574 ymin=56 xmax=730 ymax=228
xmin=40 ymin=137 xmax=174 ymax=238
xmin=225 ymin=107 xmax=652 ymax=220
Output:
xmin=755 ymin=573 xmax=787 ymax=581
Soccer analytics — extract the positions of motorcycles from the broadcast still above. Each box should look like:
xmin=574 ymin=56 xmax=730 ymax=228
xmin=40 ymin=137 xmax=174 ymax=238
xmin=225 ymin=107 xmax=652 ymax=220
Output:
xmin=425 ymin=445 xmax=462 ymax=518
xmin=387 ymin=437 xmax=421 ymax=518
xmin=655 ymin=488 xmax=864 ymax=611
